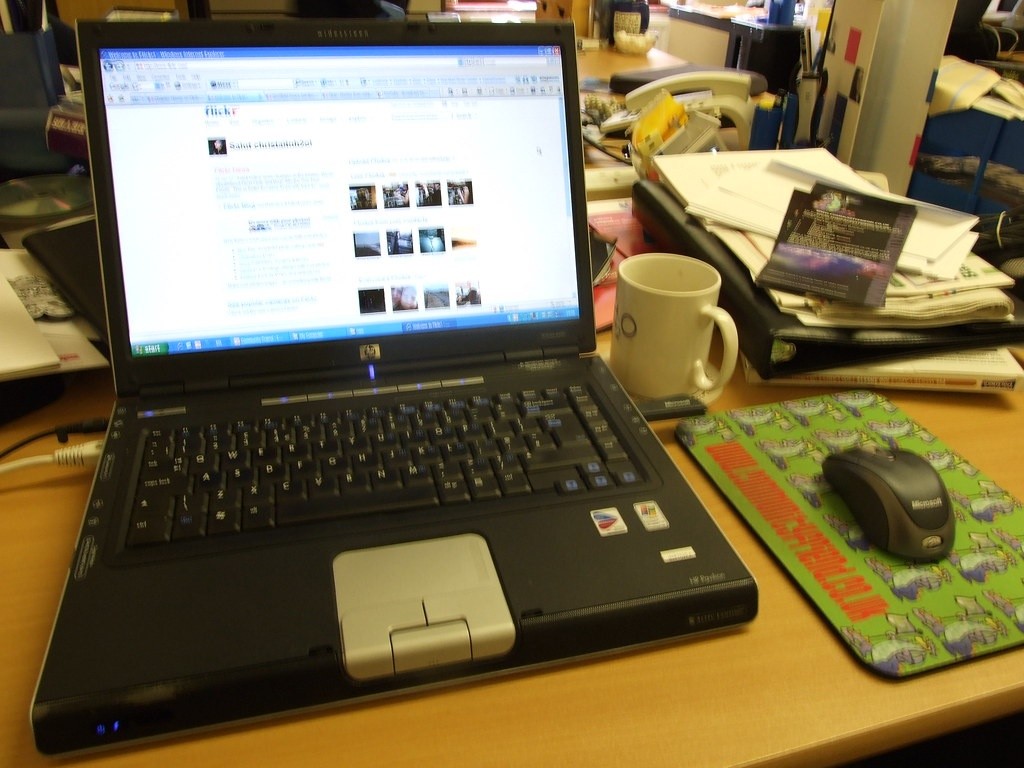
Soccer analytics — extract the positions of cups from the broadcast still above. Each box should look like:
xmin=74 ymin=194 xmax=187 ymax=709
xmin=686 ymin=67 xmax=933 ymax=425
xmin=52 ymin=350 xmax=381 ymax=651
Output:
xmin=607 ymin=253 xmax=739 ymax=401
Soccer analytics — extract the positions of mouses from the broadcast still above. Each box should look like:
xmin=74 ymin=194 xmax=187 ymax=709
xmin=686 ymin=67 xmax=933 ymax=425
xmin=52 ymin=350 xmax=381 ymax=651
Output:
xmin=822 ymin=446 xmax=956 ymax=561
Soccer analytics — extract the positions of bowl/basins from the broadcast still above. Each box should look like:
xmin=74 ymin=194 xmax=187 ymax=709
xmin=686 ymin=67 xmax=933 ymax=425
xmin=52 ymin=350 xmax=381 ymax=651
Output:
xmin=614 ymin=31 xmax=657 ymax=55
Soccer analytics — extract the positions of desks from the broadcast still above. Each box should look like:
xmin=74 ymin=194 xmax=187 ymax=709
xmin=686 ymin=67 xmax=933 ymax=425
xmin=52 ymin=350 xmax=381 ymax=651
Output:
xmin=662 ymin=7 xmax=804 ymax=70
xmin=0 ymin=47 xmax=1023 ymax=768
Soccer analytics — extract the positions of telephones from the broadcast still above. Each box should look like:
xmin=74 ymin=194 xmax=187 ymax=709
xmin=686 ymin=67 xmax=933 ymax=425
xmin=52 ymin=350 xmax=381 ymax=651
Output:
xmin=600 ymin=71 xmax=756 ymax=151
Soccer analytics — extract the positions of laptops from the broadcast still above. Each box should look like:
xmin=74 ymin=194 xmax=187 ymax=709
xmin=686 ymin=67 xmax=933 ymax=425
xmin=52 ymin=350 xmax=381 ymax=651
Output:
xmin=29 ymin=16 xmax=759 ymax=759
